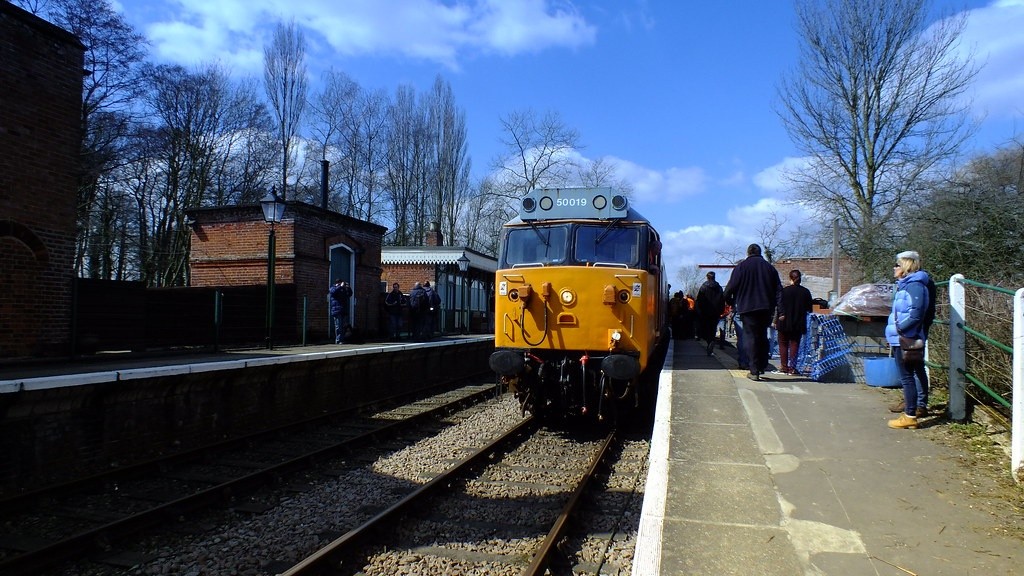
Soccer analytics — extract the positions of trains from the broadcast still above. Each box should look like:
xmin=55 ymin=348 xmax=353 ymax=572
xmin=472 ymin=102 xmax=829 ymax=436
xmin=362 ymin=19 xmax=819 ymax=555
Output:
xmin=488 ymin=187 xmax=666 ymax=434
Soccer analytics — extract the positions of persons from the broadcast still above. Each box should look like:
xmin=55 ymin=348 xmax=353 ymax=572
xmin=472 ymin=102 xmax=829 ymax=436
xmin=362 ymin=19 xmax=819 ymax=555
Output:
xmin=777 ymin=270 xmax=813 ymax=375
xmin=650 ymin=240 xmax=662 ymax=265
xmin=697 ymin=271 xmax=724 ymax=355
xmin=724 ymin=243 xmax=781 ymax=380
xmin=410 ymin=280 xmax=441 ymax=340
xmin=732 ymin=309 xmax=778 ymax=369
xmin=884 ymin=250 xmax=936 ymax=430
xmin=669 ymin=291 xmax=698 ymax=341
xmin=329 ymin=278 xmax=353 ymax=344
xmin=385 ymin=283 xmax=406 ymax=341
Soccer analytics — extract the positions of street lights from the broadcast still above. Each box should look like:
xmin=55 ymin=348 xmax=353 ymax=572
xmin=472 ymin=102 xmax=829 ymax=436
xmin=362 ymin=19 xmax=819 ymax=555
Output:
xmin=260 ymin=184 xmax=289 ymax=349
xmin=456 ymin=250 xmax=471 ymax=335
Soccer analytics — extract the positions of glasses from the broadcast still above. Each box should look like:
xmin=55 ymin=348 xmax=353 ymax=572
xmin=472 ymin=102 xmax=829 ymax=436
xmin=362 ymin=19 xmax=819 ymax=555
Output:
xmin=896 ymin=264 xmax=901 ymax=267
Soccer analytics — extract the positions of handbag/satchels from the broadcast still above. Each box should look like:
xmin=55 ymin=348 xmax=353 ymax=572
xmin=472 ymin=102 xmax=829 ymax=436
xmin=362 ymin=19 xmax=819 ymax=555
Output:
xmin=898 ymin=335 xmax=924 ymax=361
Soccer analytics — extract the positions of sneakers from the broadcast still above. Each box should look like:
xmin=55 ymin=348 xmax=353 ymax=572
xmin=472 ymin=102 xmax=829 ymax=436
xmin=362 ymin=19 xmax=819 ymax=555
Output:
xmin=889 ymin=412 xmax=918 ymax=430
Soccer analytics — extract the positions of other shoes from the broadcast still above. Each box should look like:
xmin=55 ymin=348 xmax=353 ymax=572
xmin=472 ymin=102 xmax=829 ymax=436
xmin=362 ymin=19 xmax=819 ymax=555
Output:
xmin=889 ymin=403 xmax=905 ymax=412
xmin=771 ymin=369 xmax=788 ymax=375
xmin=917 ymin=408 xmax=927 ymax=417
xmin=788 ymin=370 xmax=800 ymax=376
xmin=748 ymin=372 xmax=758 ymax=381
xmin=707 ymin=341 xmax=715 ymax=355
